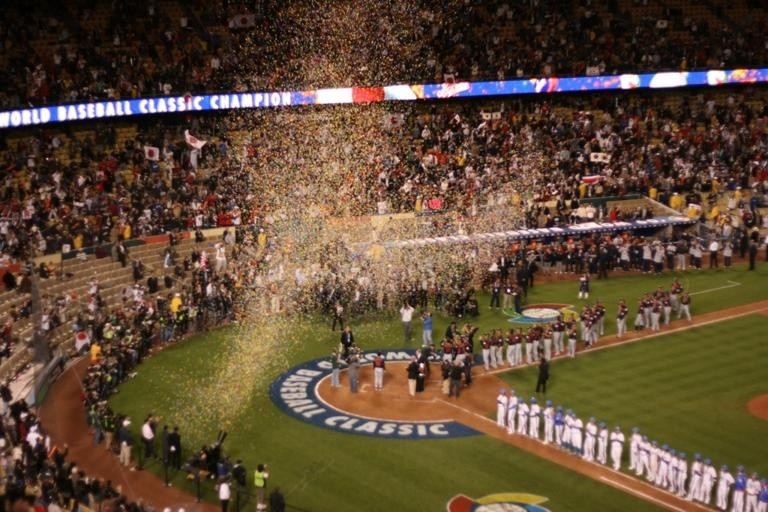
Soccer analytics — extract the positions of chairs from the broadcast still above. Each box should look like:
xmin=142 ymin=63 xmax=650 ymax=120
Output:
xmin=0 ymin=233 xmax=251 ymax=389
